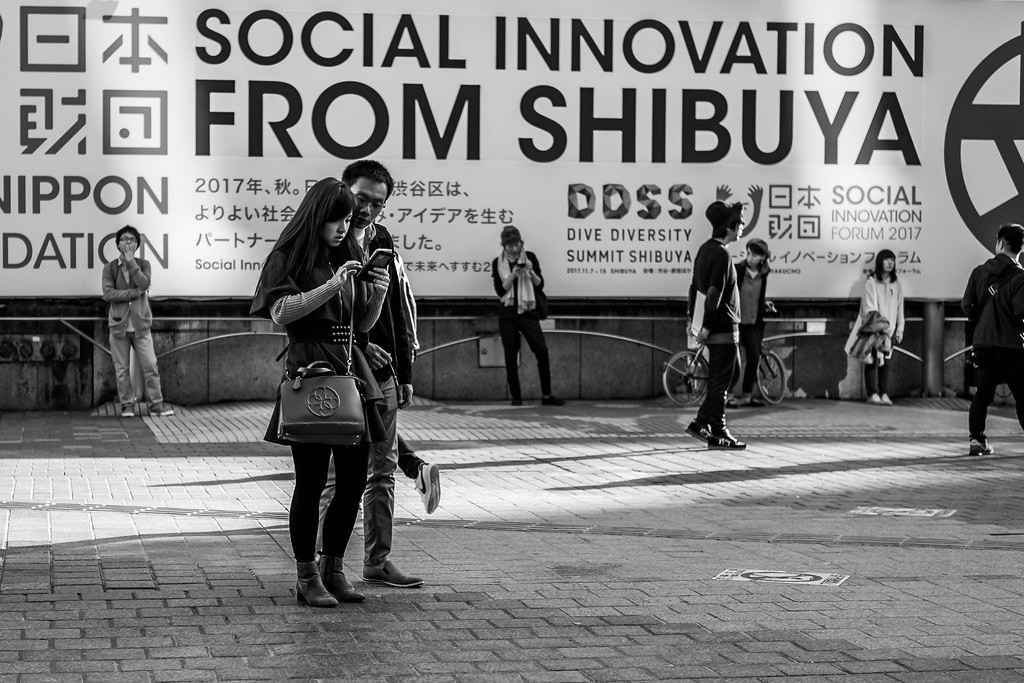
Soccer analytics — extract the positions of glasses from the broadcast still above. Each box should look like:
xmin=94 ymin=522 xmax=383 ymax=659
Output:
xmin=356 ymin=195 xmax=386 ymax=209
xmin=119 ymin=237 xmax=136 ymax=242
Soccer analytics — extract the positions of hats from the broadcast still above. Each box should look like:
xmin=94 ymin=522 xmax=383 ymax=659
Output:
xmin=501 ymin=226 xmax=521 ymax=246
xmin=705 ymin=201 xmax=743 ymax=238
xmin=114 ymin=224 xmax=140 ymax=251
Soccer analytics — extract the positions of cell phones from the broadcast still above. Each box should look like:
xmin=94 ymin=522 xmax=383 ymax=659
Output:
xmin=357 ymin=249 xmax=394 ymax=283
xmin=518 ymin=263 xmax=526 ymax=268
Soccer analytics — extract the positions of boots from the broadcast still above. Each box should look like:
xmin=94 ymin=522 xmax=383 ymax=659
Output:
xmin=317 ymin=550 xmax=365 ymax=602
xmin=295 ymin=561 xmax=337 ymax=607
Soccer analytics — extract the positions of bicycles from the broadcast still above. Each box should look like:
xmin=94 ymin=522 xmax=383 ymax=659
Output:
xmin=661 ymin=344 xmax=786 ymax=407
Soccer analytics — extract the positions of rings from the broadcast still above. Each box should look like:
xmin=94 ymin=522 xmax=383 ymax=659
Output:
xmin=382 ymin=276 xmax=385 ymax=280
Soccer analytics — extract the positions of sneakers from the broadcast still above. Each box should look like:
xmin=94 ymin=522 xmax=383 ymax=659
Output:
xmin=708 ymin=429 xmax=746 ymax=450
xmin=414 ymin=462 xmax=441 ymax=514
xmin=685 ymin=421 xmax=711 ymax=442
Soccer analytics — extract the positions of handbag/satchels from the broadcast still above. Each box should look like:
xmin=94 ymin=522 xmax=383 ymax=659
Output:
xmin=535 ymin=288 xmax=550 ymax=320
xmin=277 ymin=375 xmax=366 ymax=445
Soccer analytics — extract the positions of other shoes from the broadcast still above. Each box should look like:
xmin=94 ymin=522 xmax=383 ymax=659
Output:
xmin=362 ymin=559 xmax=424 ymax=587
xmin=512 ymin=398 xmax=522 ymax=406
xmin=540 ymin=395 xmax=566 ymax=407
xmin=880 ymin=392 xmax=893 ymax=406
xmin=969 ymin=438 xmax=993 ymax=456
xmin=867 ymin=393 xmax=881 ymax=405
xmin=150 ymin=403 xmax=175 ymax=416
xmin=725 ymin=398 xmax=738 ymax=409
xmin=122 ymin=406 xmax=134 ymax=417
xmin=740 ymin=398 xmax=765 ymax=407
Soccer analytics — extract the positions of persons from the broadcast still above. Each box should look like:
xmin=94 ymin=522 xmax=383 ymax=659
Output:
xmin=961 ymin=223 xmax=1024 ymax=455
xmin=685 ymin=201 xmax=747 ymax=449
xmin=316 ymin=160 xmax=441 ymax=586
xmin=846 ymin=249 xmax=905 ymax=405
xmin=492 ymin=226 xmax=565 ymax=406
xmin=249 ymin=176 xmax=390 ymax=608
xmin=102 ymin=225 xmax=175 ymax=417
xmin=725 ymin=238 xmax=770 ymax=408
